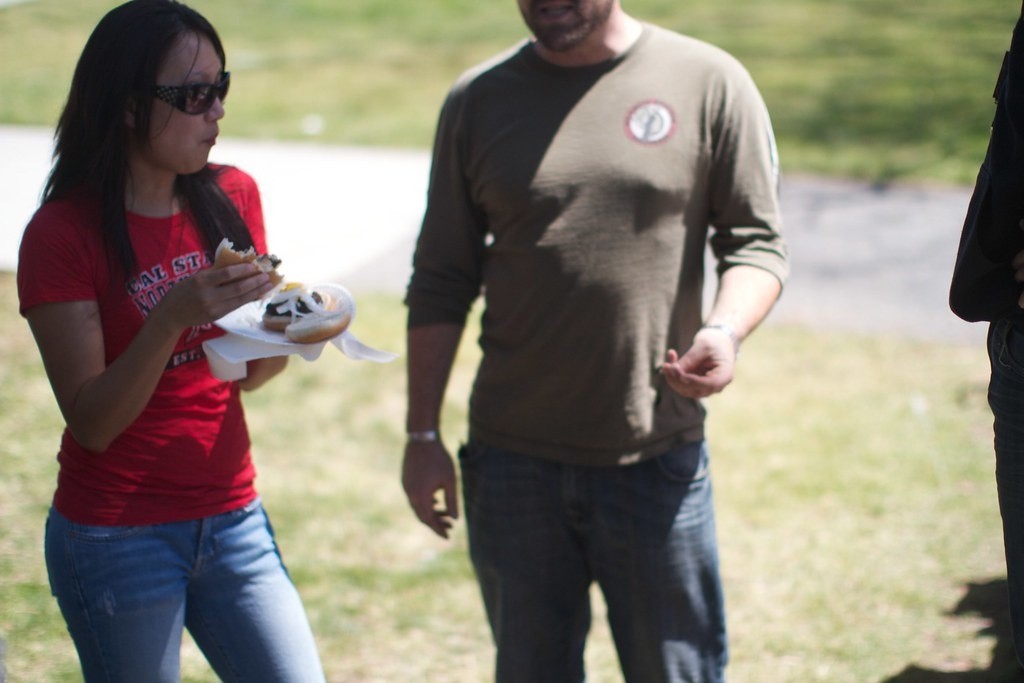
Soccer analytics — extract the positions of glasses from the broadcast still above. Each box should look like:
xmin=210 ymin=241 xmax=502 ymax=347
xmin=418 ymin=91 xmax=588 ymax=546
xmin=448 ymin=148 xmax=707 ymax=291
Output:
xmin=151 ymin=71 xmax=230 ymax=115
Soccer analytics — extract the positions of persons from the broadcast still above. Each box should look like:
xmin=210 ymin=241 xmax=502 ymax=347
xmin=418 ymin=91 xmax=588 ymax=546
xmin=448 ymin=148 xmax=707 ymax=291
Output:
xmin=401 ymin=1 xmax=792 ymax=682
xmin=949 ymin=0 xmax=1024 ymax=668
xmin=16 ymin=0 xmax=317 ymax=683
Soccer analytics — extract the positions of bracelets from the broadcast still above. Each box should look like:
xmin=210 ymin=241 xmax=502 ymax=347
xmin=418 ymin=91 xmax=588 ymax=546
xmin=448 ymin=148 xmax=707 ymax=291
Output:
xmin=407 ymin=429 xmax=439 ymax=443
xmin=701 ymin=324 xmax=739 ymax=356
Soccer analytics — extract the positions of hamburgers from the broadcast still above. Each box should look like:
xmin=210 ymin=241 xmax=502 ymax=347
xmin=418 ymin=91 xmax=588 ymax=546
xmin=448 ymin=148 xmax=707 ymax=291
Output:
xmin=215 ymin=238 xmax=284 ymax=290
xmin=262 ymin=282 xmax=351 ymax=342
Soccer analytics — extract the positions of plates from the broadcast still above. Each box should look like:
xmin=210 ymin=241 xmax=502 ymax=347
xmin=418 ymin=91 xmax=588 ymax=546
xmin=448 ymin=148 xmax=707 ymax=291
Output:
xmin=214 ymin=300 xmax=325 ymax=347
